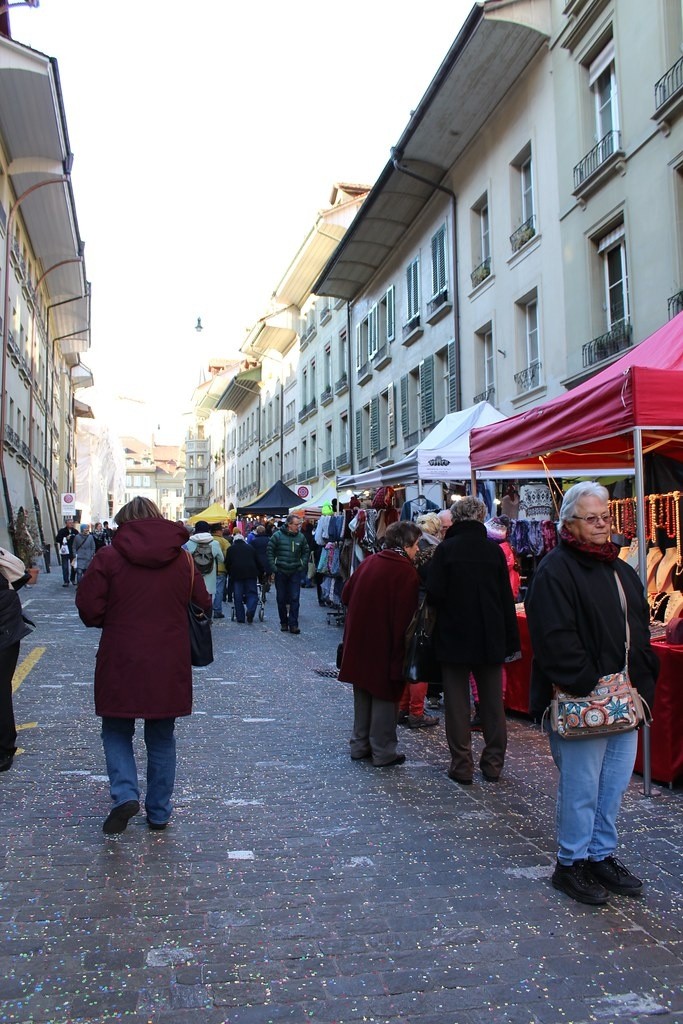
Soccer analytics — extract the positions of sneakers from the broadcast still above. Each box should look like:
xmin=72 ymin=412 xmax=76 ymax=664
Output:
xmin=582 ymin=853 xmax=643 ymax=895
xmin=552 ymin=860 xmax=610 ymax=903
xmin=398 ymin=711 xmax=440 ymax=728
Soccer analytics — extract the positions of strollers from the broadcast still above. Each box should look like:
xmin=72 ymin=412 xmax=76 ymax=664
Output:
xmin=229 ymin=579 xmax=266 ymax=622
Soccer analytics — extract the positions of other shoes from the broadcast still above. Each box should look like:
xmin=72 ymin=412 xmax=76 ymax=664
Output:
xmin=427 ymin=696 xmax=440 ymax=709
xmin=103 ymin=800 xmax=140 ymax=834
xmin=63 ymin=581 xmax=69 ymax=587
xmin=71 ymin=579 xmax=77 ymax=585
xmin=449 ymin=773 xmax=474 ymax=784
xmin=375 ymin=755 xmax=405 ymax=767
xmin=281 ymin=623 xmax=288 ymax=631
xmin=146 ymin=816 xmax=166 ymax=830
xmin=246 ymin=611 xmax=253 ymax=623
xmin=0 ymin=752 xmax=15 ymax=772
xmin=289 ymin=625 xmax=300 ymax=633
xmin=486 ymin=774 xmax=499 ymax=781
xmin=214 ymin=613 xmax=224 ymax=617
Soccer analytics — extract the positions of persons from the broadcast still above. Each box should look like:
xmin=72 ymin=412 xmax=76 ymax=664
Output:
xmin=0 ymin=573 xmax=37 ymax=773
xmin=524 ymin=479 xmax=660 ymax=904
xmin=209 ymin=523 xmax=266 ymax=624
xmin=75 ymin=496 xmax=213 ymax=835
xmin=266 ymin=515 xmax=310 ymax=634
xmin=337 ymin=495 xmax=518 ymax=786
xmin=72 ymin=524 xmax=95 ymax=586
xmin=55 ymin=519 xmax=80 ymax=587
xmin=272 ymin=521 xmax=283 ymax=534
xmin=103 ymin=521 xmax=114 ymax=545
xmin=184 ymin=521 xmax=224 ymax=624
xmin=246 ymin=524 xmax=272 ymax=592
xmin=90 ymin=522 xmax=110 ymax=552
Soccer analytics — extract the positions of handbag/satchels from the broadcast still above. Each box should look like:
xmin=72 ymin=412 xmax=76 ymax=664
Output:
xmin=184 ymin=548 xmax=214 ymax=667
xmin=0 ymin=547 xmax=27 ymax=590
xmin=59 ymin=537 xmax=69 ymax=555
xmin=541 ymin=672 xmax=653 ymax=740
xmin=410 ymin=599 xmax=437 ymax=682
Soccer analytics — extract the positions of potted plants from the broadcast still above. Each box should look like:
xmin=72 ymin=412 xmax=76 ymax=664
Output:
xmin=6 ymin=501 xmax=45 ymax=585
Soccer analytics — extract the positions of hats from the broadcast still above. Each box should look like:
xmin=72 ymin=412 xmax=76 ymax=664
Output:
xmin=232 ymin=527 xmax=241 ymax=534
xmin=484 ymin=514 xmax=509 ymax=543
xmin=210 ymin=523 xmax=225 ymax=530
xmin=194 ymin=521 xmax=209 ymax=532
xmin=80 ymin=524 xmax=88 ymax=531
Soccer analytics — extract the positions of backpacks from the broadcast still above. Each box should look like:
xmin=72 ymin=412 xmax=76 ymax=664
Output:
xmin=192 ymin=540 xmax=213 ymax=573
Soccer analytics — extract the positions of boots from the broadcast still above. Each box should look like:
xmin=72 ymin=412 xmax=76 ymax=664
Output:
xmin=471 ymin=702 xmax=483 ymax=733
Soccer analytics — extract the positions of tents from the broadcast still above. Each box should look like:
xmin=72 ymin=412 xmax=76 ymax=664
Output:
xmin=470 ymin=309 xmax=683 ymax=796
xmin=336 ymin=398 xmax=509 ymax=515
xmin=237 ymin=480 xmax=307 ymax=515
xmin=289 ymin=479 xmax=368 ymax=516
xmin=186 ymin=501 xmax=229 ymax=526
xmin=228 ymin=485 xmax=270 ymax=520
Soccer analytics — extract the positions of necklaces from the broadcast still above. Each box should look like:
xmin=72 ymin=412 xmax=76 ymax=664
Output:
xmin=608 ymin=491 xmax=683 ymax=634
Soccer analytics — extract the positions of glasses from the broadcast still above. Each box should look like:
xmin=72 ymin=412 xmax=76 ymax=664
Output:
xmin=572 ymin=513 xmax=615 ymax=523
